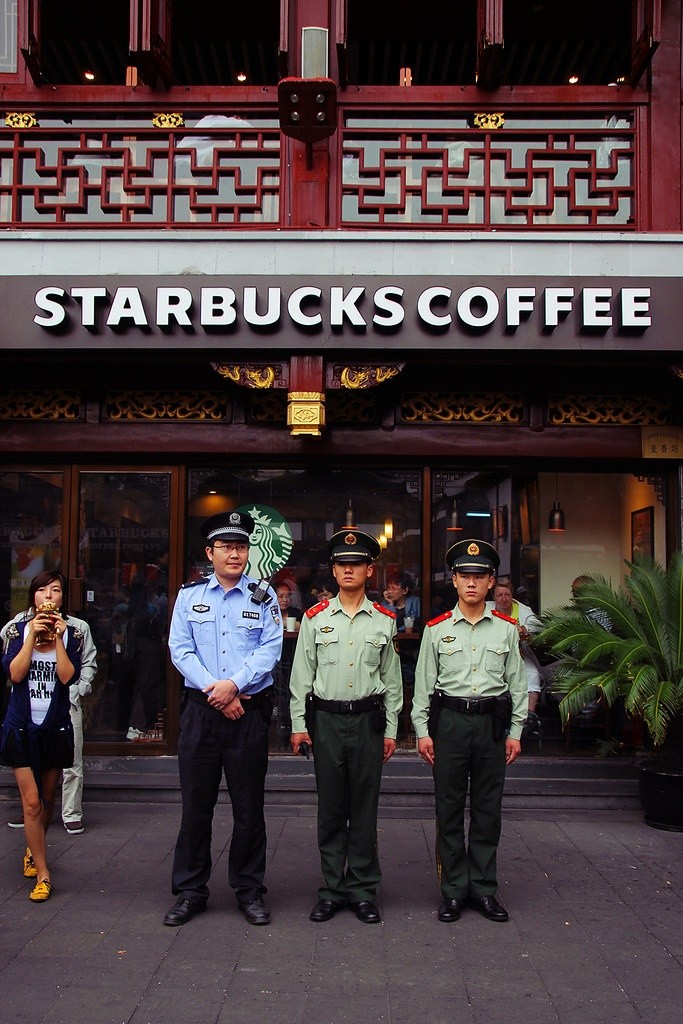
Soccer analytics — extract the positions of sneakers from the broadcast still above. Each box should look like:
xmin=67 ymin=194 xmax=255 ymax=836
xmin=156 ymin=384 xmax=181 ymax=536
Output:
xmin=163 ymin=895 xmax=206 ymax=925
xmin=238 ymin=897 xmax=270 ymax=924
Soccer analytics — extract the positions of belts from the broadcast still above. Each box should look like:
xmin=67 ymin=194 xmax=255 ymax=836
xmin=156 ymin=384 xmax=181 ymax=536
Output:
xmin=314 ymin=695 xmax=379 ymax=714
xmin=442 ymin=691 xmax=507 ymax=715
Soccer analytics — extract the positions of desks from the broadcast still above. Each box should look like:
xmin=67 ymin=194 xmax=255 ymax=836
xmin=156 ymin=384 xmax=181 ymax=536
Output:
xmin=283 ymin=629 xmax=420 ymax=641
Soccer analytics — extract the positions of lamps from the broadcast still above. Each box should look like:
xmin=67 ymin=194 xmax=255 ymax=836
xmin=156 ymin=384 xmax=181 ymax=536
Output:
xmin=379 ymin=513 xmax=393 ymax=550
xmin=548 ymin=472 xmax=566 ymax=532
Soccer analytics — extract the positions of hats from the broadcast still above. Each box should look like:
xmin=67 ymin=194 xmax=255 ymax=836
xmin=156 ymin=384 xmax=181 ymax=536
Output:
xmin=200 ymin=511 xmax=254 ymax=545
xmin=326 ymin=529 xmax=381 ymax=564
xmin=445 ymin=538 xmax=500 ymax=576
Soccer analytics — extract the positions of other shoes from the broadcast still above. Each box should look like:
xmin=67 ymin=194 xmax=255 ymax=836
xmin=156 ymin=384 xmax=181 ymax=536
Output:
xmin=30 ymin=879 xmax=54 ymax=903
xmin=64 ymin=821 xmax=84 ymax=835
xmin=8 ymin=814 xmax=25 ymax=828
xmin=23 ymin=847 xmax=37 ymax=877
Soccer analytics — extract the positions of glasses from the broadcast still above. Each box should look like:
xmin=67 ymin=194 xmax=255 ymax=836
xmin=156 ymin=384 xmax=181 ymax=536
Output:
xmin=211 ymin=544 xmax=249 ymax=553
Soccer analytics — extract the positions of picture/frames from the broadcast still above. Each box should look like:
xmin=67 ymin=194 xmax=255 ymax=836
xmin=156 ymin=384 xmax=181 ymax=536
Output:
xmin=446 ymin=486 xmax=490 ymax=531
xmin=631 ymin=505 xmax=654 ymax=565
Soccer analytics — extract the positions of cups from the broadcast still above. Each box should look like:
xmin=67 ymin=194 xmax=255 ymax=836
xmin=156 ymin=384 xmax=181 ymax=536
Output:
xmin=404 ymin=617 xmax=414 ymax=633
xmin=286 ymin=617 xmax=296 ymax=632
xmin=35 ymin=610 xmax=58 ymax=645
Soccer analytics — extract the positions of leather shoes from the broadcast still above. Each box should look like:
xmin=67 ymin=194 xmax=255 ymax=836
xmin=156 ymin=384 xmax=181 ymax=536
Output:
xmin=310 ymin=899 xmax=340 ymax=921
xmin=467 ymin=895 xmax=509 ymax=921
xmin=438 ymin=897 xmax=467 ymax=921
xmin=347 ymin=900 xmax=380 ymax=922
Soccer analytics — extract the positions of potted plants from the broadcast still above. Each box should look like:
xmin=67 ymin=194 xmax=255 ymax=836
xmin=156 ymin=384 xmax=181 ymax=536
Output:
xmin=528 ymin=550 xmax=683 ymax=831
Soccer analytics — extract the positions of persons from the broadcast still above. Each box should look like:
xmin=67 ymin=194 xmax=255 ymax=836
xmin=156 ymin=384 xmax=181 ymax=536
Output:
xmin=410 ymin=540 xmax=530 ymax=922
xmin=491 ymin=576 xmax=545 ymax=713
xmin=175 ymin=103 xmax=257 ymax=176
xmin=381 ymin=571 xmax=420 ymax=630
xmin=0 ymin=570 xmax=98 ymax=903
xmin=570 ymin=574 xmax=613 ymax=633
xmin=311 ymin=579 xmax=335 ymax=603
xmin=290 ymin=531 xmax=405 ymax=924
xmin=163 ymin=511 xmax=283 ymax=927
xmin=272 ymin=579 xmax=305 ymax=630
xmin=76 ymin=569 xmax=168 ymax=718
